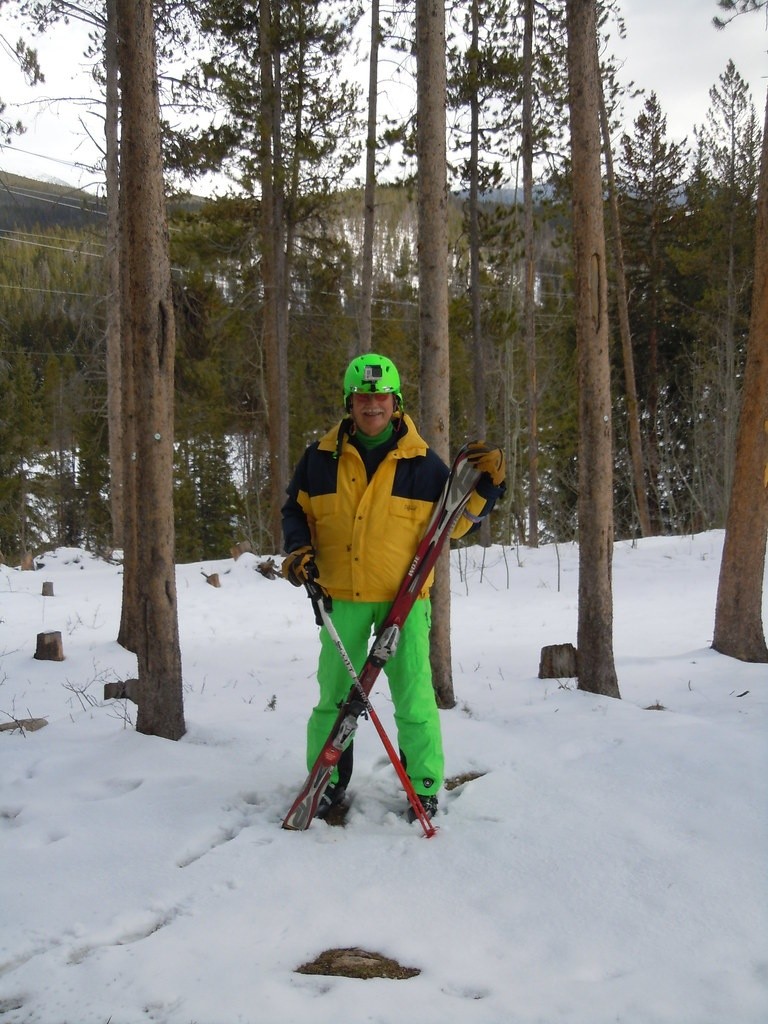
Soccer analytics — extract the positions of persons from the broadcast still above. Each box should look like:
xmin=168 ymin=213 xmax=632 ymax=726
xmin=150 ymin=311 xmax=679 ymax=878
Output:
xmin=280 ymin=354 xmax=508 ymax=823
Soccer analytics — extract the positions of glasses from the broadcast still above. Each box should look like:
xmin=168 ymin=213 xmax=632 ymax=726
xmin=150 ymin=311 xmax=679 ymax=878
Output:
xmin=352 ymin=393 xmax=394 ymax=402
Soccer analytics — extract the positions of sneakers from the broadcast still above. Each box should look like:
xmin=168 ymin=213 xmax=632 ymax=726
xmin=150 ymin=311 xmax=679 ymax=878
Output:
xmin=313 ymin=779 xmax=345 ymax=819
xmin=407 ymin=794 xmax=438 ymax=824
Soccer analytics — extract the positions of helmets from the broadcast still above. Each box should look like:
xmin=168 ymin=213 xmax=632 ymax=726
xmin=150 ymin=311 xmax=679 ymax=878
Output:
xmin=342 ymin=354 xmax=400 ymax=407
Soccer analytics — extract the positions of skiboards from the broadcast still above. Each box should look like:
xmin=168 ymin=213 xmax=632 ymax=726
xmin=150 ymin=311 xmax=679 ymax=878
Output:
xmin=280 ymin=437 xmax=485 ymax=831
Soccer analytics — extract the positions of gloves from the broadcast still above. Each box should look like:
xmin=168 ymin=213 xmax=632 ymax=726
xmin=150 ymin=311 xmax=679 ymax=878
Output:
xmin=287 ymin=550 xmax=320 ymax=587
xmin=465 ymin=440 xmax=506 ymax=486
